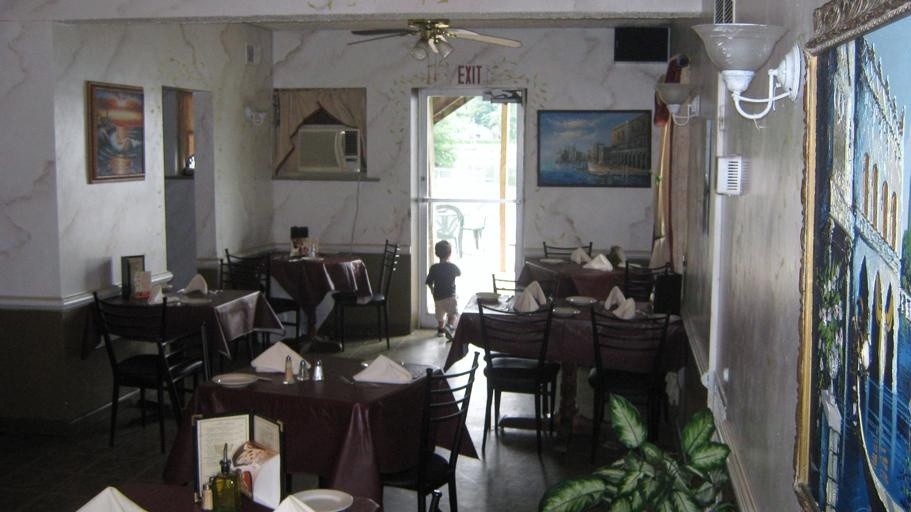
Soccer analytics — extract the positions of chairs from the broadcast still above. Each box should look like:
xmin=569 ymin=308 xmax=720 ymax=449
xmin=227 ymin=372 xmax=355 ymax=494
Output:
xmin=433 ymin=203 xmax=463 ymax=259
xmin=92 ymin=291 xmax=205 ymax=448
xmin=476 ymin=296 xmax=560 ymax=456
xmin=330 ymin=239 xmax=400 ymax=352
xmin=543 ymin=241 xmax=593 ymax=258
xmin=623 ymin=260 xmax=673 ymax=303
xmin=492 ymin=274 xmax=559 ymax=303
xmin=376 ymin=350 xmax=479 ymax=512
xmin=587 ymin=303 xmax=668 ymax=465
xmin=225 ymin=248 xmax=300 ymax=349
xmin=219 ymin=258 xmax=265 ymax=364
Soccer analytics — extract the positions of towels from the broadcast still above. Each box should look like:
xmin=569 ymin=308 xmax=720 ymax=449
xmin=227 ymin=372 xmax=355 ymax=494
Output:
xmin=604 ymin=285 xmax=627 ymax=311
xmin=526 ymin=280 xmax=547 ymax=305
xmin=76 ymin=485 xmax=146 ymax=512
xmin=513 ymin=287 xmax=540 ymax=313
xmin=250 ymin=340 xmax=311 ymax=376
xmin=570 ymin=246 xmax=592 ymax=264
xmin=612 ymin=296 xmax=636 ymax=320
xmin=581 ymin=252 xmax=613 ymax=272
xmin=273 ymin=492 xmax=313 ymax=512
xmin=352 ymin=354 xmax=413 ymax=386
xmin=147 ymin=285 xmax=180 ymax=306
xmin=177 ymin=274 xmax=208 ymax=296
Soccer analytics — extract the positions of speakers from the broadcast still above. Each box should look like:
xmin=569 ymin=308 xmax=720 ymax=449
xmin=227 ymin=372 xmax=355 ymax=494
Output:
xmin=614 ymin=28 xmax=668 ymax=61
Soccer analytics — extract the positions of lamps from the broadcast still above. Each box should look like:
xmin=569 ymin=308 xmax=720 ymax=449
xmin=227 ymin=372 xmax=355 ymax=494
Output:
xmin=537 ymin=109 xmax=652 ymax=188
xmin=410 ymin=32 xmax=454 ymax=85
xmin=690 ymin=22 xmax=806 ymax=120
xmin=84 ymin=80 xmax=145 ymax=184
xmin=652 ymin=82 xmax=702 ymax=127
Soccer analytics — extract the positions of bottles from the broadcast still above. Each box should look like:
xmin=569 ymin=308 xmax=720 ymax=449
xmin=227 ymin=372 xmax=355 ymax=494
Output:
xmin=284 ymin=357 xmax=325 ymax=384
xmin=201 ymin=441 xmax=243 ymax=511
xmin=308 ymin=243 xmax=320 ymax=257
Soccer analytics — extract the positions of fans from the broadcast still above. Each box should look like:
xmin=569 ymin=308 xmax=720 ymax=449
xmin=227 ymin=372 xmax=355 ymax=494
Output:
xmin=346 ymin=15 xmax=521 ymax=61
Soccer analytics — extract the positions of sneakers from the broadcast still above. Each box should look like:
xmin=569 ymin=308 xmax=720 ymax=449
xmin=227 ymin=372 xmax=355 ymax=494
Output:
xmin=436 ymin=323 xmax=456 ymax=341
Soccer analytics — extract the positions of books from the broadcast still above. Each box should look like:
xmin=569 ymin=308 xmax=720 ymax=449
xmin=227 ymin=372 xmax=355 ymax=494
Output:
xmin=121 ymin=256 xmax=144 ymax=299
xmin=191 ymin=409 xmax=286 ymax=512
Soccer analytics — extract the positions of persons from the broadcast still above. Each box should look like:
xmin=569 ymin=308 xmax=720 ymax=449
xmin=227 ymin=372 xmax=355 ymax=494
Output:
xmin=426 ymin=241 xmax=460 ymax=339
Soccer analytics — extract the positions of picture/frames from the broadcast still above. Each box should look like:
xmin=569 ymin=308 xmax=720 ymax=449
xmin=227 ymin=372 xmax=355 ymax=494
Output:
xmin=792 ymin=0 xmax=911 ymax=512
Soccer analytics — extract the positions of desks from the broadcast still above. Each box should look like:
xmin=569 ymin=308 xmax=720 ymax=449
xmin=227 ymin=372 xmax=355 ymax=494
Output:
xmin=462 ymin=216 xmax=484 ymax=250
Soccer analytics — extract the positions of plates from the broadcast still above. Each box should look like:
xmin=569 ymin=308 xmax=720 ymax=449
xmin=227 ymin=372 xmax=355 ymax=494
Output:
xmin=360 ymin=357 xmax=407 ymax=370
xmin=539 ymin=258 xmax=564 ymax=265
xmin=550 ymin=307 xmax=583 ymax=318
xmin=475 ymin=292 xmax=500 ymax=302
xmin=617 ymin=262 xmax=643 ymax=270
xmin=289 ymin=483 xmax=354 ymax=512
xmin=301 ymin=256 xmax=324 ymax=262
xmin=212 ymin=372 xmax=258 ymax=390
xmin=565 ymin=295 xmax=598 ymax=307
xmin=180 ymin=296 xmax=215 ymax=305
